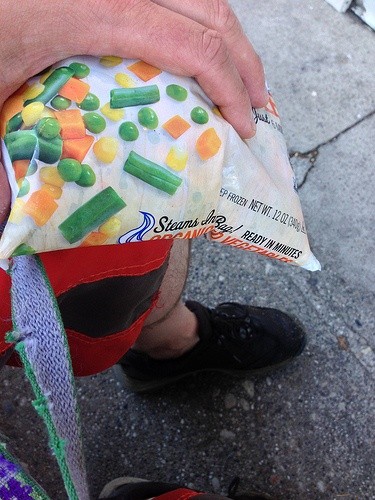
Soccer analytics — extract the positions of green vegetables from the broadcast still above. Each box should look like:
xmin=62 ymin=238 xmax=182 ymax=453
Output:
xmin=3 ymin=55 xmax=225 ymax=243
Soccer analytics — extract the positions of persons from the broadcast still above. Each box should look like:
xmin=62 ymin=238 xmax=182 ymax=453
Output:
xmin=0 ymin=0 xmax=307 ymax=500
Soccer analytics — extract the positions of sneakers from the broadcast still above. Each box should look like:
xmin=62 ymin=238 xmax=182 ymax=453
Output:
xmin=128 ymin=301 xmax=307 ymax=396
xmin=98 ymin=476 xmax=209 ymax=500
